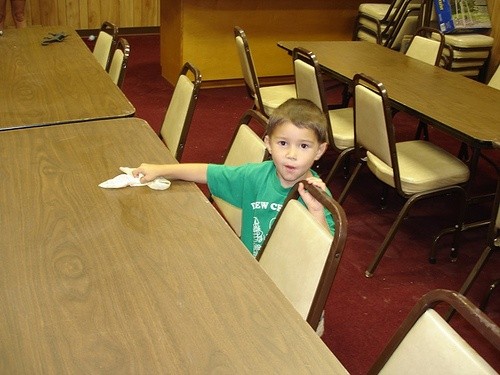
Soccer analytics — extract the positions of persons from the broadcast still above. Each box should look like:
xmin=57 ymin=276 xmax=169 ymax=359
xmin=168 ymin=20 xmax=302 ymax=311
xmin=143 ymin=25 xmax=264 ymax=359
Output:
xmin=131 ymin=98 xmax=335 ymax=338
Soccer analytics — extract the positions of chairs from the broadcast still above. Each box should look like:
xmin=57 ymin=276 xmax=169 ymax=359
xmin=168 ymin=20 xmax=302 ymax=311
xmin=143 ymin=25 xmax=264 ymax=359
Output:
xmin=92 ymin=21 xmax=119 ymax=71
xmin=158 ymin=62 xmax=202 ymax=163
xmin=108 ymin=37 xmax=130 ymax=86
xmin=208 ymin=0 xmax=500 ymax=375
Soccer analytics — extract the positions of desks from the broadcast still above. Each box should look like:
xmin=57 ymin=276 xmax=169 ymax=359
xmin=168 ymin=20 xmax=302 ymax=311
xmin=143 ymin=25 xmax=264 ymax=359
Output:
xmin=277 ymin=41 xmax=500 ymax=264
xmin=0 ymin=28 xmax=351 ymax=375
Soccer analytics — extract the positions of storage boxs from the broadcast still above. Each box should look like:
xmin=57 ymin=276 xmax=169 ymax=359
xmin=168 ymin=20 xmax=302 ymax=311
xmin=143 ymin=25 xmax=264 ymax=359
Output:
xmin=434 ymin=0 xmax=492 ymax=35
